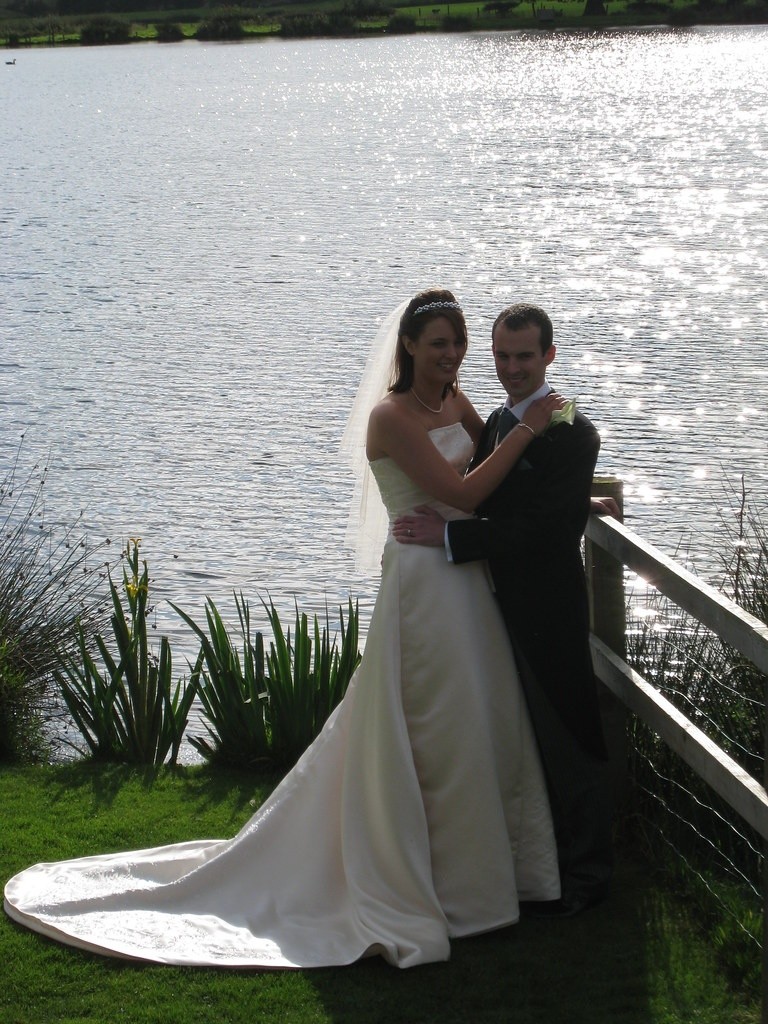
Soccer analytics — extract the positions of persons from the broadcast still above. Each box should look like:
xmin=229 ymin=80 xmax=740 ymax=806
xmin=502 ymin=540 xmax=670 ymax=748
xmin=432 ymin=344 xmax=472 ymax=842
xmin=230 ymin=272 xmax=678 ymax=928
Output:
xmin=341 ymin=291 xmax=622 ymax=969
xmin=392 ymin=303 xmax=616 ymax=920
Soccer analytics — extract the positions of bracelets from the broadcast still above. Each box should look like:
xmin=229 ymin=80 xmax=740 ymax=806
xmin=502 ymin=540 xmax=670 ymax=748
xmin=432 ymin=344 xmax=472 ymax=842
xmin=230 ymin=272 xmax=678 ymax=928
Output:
xmin=518 ymin=422 xmax=536 ymax=436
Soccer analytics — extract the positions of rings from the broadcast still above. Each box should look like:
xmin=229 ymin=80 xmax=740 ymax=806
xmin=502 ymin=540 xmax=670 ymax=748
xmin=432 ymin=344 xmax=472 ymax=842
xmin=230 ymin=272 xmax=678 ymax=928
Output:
xmin=408 ymin=529 xmax=411 ymax=536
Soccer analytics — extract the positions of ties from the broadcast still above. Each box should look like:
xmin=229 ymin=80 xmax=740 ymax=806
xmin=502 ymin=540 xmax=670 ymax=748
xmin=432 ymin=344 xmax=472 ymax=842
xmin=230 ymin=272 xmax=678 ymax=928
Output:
xmin=497 ymin=409 xmax=517 ymax=447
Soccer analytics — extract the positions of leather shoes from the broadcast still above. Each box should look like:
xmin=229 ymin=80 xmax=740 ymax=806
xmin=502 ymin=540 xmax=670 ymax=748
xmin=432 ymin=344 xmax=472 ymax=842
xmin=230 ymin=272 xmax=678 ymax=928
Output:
xmin=526 ymin=890 xmax=604 ymax=922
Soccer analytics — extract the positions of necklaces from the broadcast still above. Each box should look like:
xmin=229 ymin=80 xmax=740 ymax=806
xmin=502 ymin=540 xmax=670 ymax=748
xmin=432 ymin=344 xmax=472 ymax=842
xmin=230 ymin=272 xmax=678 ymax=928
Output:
xmin=409 ymin=385 xmax=443 ymax=412
